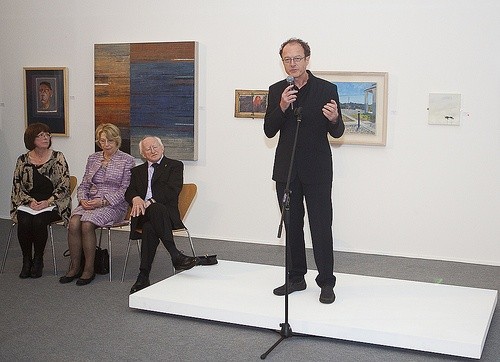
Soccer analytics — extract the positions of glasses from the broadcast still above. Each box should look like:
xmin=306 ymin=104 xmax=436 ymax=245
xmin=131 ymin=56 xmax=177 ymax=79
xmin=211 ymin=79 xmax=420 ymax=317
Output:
xmin=283 ymin=56 xmax=308 ymax=63
xmin=34 ymin=133 xmax=52 ymax=139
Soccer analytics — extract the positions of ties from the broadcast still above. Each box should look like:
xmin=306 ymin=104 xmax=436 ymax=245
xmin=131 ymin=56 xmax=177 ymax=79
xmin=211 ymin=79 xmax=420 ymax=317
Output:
xmin=151 ymin=163 xmax=157 ymax=172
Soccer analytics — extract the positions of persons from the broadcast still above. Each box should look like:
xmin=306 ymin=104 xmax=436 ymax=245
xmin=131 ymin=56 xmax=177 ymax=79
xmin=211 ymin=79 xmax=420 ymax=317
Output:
xmin=263 ymin=38 xmax=345 ymax=304
xmin=124 ymin=136 xmax=201 ymax=294
xmin=9 ymin=123 xmax=71 ymax=279
xmin=59 ymin=123 xmax=135 ymax=286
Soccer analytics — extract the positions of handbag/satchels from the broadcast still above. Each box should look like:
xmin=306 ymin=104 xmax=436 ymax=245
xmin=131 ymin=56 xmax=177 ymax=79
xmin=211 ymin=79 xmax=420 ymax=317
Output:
xmin=63 ymin=247 xmax=109 ymax=275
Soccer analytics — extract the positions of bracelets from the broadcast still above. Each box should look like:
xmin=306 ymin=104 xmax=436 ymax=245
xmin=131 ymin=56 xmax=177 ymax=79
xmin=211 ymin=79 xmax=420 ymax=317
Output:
xmin=147 ymin=198 xmax=154 ymax=204
xmin=101 ymin=200 xmax=105 ymax=207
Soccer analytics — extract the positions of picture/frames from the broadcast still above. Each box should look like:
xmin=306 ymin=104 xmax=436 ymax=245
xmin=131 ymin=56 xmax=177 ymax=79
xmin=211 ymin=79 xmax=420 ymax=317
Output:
xmin=22 ymin=67 xmax=69 ymax=137
xmin=235 ymin=90 xmax=269 ymax=118
xmin=311 ymin=71 xmax=388 ymax=147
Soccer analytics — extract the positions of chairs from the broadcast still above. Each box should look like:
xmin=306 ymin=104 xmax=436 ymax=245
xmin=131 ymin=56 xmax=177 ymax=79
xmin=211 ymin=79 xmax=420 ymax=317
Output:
xmin=1 ymin=176 xmax=77 ymax=278
xmin=77 ymin=201 xmax=132 ymax=282
xmin=121 ymin=184 xmax=198 ymax=281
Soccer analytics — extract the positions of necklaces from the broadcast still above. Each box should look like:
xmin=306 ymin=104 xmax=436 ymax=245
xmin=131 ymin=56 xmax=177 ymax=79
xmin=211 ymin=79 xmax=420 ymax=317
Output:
xmin=105 ymin=155 xmax=110 ymax=161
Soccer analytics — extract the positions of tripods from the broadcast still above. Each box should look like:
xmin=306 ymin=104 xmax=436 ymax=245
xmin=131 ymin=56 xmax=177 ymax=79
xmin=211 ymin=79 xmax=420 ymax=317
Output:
xmin=260 ymin=111 xmax=319 ymax=360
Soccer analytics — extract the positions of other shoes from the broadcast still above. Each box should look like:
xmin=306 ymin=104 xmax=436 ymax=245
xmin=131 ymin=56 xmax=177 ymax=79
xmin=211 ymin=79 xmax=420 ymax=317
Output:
xmin=76 ymin=274 xmax=96 ymax=286
xmin=20 ymin=258 xmax=32 ymax=277
xmin=60 ymin=271 xmax=83 ymax=283
xmin=29 ymin=258 xmax=43 ymax=278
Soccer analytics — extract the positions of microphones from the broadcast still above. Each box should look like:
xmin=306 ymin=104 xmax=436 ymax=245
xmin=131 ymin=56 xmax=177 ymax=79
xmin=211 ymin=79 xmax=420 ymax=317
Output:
xmin=286 ymin=75 xmax=295 ymax=111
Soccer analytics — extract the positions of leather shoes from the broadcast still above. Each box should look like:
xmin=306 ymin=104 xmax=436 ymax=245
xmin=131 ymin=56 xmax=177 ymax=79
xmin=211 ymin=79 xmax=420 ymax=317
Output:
xmin=172 ymin=251 xmax=200 ymax=270
xmin=319 ymin=285 xmax=335 ymax=303
xmin=130 ymin=275 xmax=149 ymax=292
xmin=273 ymin=279 xmax=306 ymax=295
